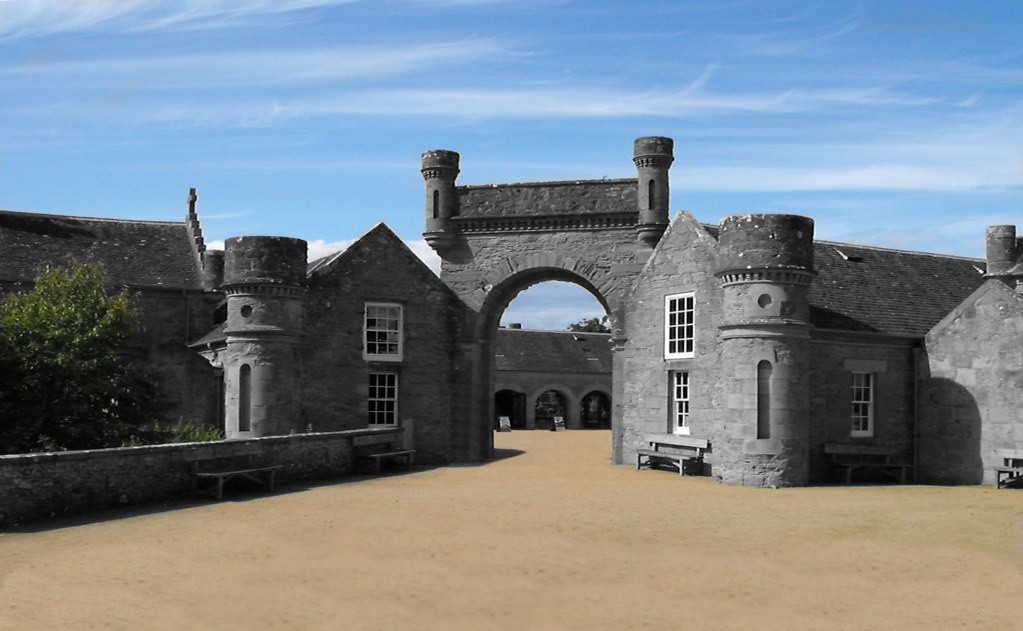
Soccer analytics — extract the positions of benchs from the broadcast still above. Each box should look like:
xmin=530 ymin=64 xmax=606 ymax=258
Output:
xmin=350 ymin=443 xmax=416 ymax=478
xmin=631 ymin=434 xmax=709 ymax=477
xmin=190 ymin=454 xmax=290 ymax=502
xmin=997 ymin=457 xmax=1023 ymax=489
xmin=822 ymin=442 xmax=912 ymax=485
